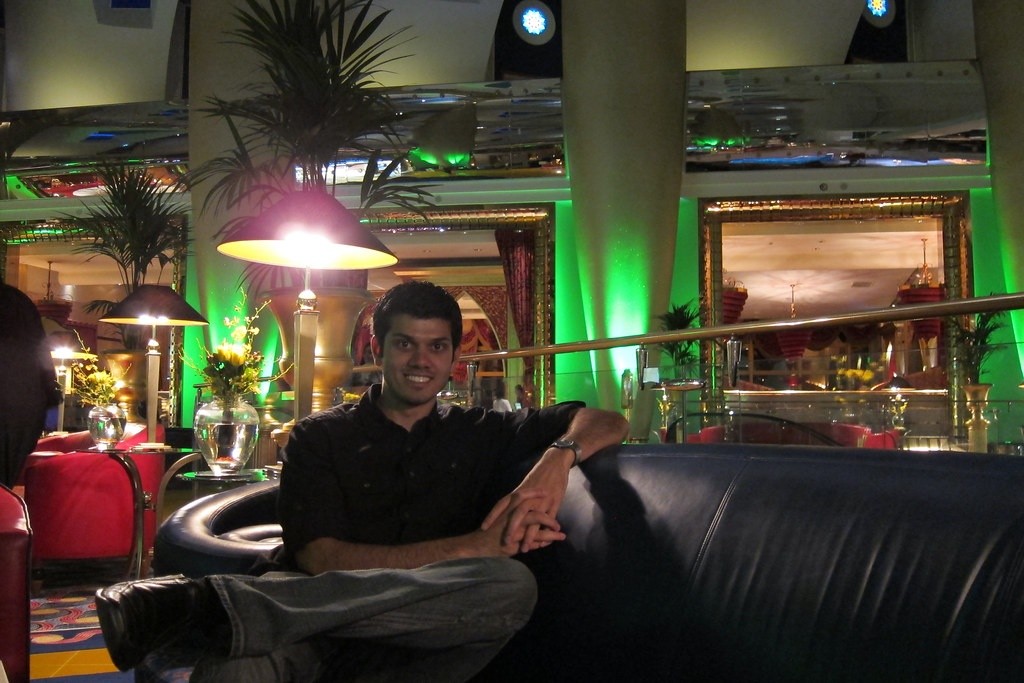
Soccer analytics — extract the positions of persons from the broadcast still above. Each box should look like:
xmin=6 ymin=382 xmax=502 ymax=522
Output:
xmin=94 ymin=283 xmax=630 ymax=683
xmin=515 ymin=385 xmax=522 ymax=394
xmin=0 ymin=282 xmax=58 ymax=487
xmin=356 ymin=343 xmax=381 ymax=385
xmin=493 ymin=392 xmax=512 ymax=412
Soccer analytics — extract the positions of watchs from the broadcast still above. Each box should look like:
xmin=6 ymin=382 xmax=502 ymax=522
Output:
xmin=551 ymin=440 xmax=581 ymax=469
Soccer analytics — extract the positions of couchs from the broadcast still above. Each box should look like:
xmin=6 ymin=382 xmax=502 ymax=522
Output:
xmin=147 ymin=439 xmax=1024 ymax=683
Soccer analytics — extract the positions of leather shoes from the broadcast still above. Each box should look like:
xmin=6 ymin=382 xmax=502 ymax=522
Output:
xmin=95 ymin=573 xmax=200 ymax=672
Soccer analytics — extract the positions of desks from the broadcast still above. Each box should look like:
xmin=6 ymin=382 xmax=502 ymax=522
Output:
xmin=172 ymin=467 xmax=273 ymax=500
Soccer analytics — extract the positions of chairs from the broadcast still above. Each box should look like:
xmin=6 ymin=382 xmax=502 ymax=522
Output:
xmin=0 ymin=419 xmax=163 ymax=683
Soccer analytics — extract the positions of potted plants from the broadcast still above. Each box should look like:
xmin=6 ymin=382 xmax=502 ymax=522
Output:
xmin=57 ymin=158 xmax=203 ymax=402
xmin=51 ymin=326 xmax=134 ymax=451
xmin=177 ymin=297 xmax=297 ymax=475
xmin=943 ymin=293 xmax=1020 ymax=456
xmin=183 ymin=0 xmax=443 ymax=417
xmin=643 ymin=295 xmax=708 ymax=447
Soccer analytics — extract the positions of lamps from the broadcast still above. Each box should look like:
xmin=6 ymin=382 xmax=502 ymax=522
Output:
xmin=218 ymin=187 xmax=400 ymax=426
xmin=97 ymin=284 xmax=209 ymax=451
